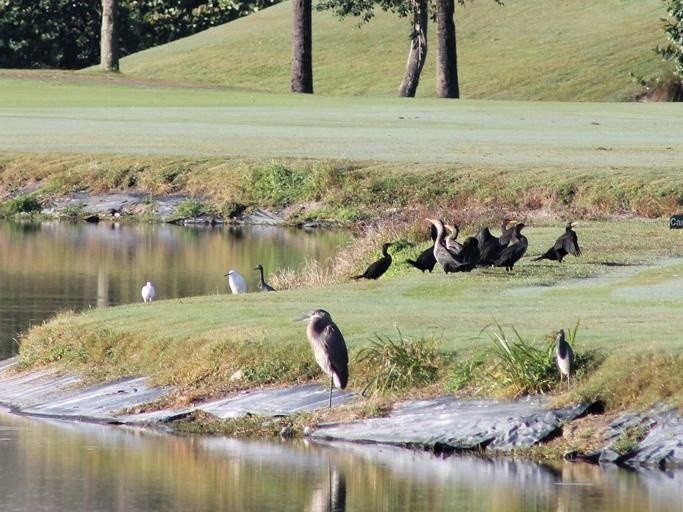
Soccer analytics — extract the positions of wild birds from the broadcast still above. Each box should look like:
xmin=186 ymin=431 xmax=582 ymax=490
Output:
xmin=140 ymin=281 xmax=154 ymax=303
xmin=254 ymin=264 xmax=275 ymax=291
xmin=530 ymin=222 xmax=582 ymax=263
xmin=349 ymin=243 xmax=395 ymax=279
xmin=223 ymin=270 xmax=246 ymax=294
xmin=403 ymin=218 xmax=527 ymax=280
xmin=554 ymin=329 xmax=573 ymax=390
xmin=292 ymin=309 xmax=348 ymax=408
